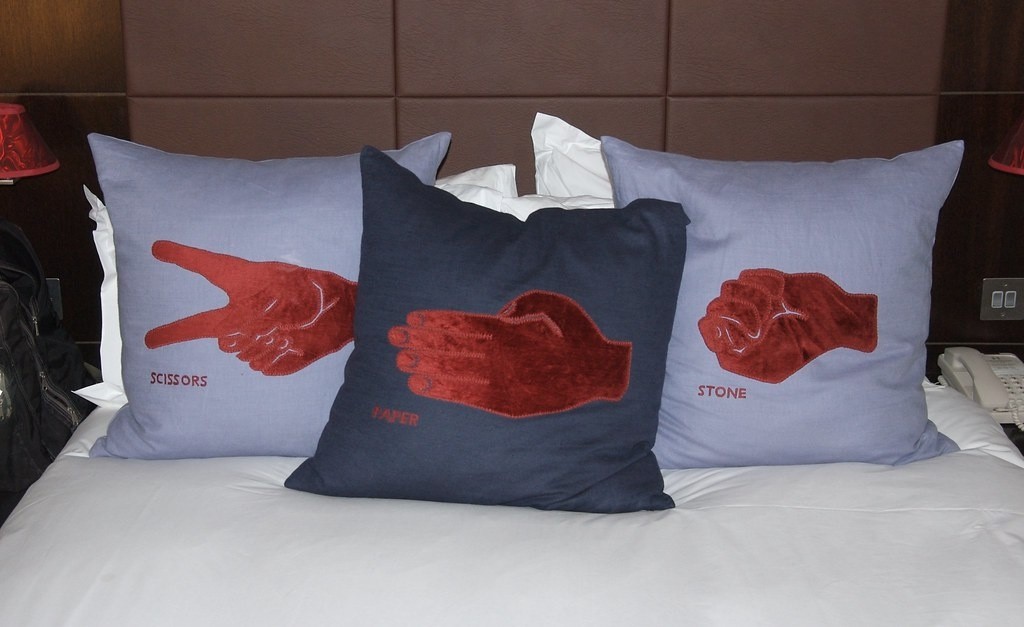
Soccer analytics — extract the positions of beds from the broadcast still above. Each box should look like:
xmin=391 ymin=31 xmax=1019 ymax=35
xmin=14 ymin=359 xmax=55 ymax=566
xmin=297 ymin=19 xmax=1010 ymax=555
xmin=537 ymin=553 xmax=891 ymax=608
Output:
xmin=0 ymin=0 xmax=1024 ymax=627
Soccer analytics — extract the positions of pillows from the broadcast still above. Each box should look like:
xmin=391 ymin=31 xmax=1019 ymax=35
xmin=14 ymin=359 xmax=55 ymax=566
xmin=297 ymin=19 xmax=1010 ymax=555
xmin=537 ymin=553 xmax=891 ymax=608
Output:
xmin=87 ymin=131 xmax=454 ymax=460
xmin=598 ymin=135 xmax=966 ymax=469
xmin=439 ymin=180 xmax=613 ymax=221
xmin=530 ymin=110 xmax=609 ymax=195
xmin=70 ymin=164 xmax=520 ymax=409
xmin=284 ymin=146 xmax=692 ymax=514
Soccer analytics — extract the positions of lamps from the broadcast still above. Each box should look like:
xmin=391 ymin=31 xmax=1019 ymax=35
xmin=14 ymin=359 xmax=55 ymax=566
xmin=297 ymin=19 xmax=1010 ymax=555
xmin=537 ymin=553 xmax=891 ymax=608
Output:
xmin=0 ymin=104 xmax=60 ymax=186
xmin=986 ymin=110 xmax=1024 ymax=173
